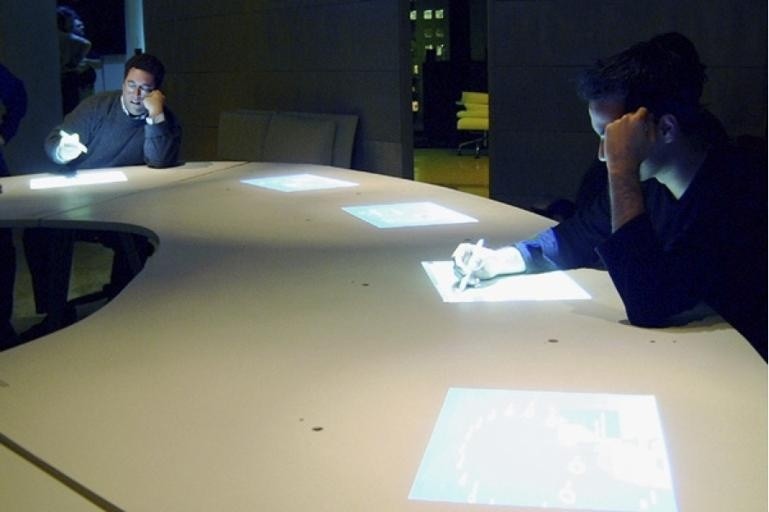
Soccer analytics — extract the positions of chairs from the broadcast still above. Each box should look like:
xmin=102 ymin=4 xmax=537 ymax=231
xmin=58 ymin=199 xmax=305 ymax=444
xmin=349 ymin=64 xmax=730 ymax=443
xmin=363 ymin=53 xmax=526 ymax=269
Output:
xmin=211 ymin=107 xmax=361 ymax=171
xmin=455 ymin=90 xmax=491 ymax=160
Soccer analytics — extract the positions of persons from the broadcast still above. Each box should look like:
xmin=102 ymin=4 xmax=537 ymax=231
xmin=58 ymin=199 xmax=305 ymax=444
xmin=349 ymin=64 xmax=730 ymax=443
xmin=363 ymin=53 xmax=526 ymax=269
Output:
xmin=72 ymin=18 xmax=104 ymax=102
xmin=0 ymin=63 xmax=29 ymax=177
xmin=451 ymin=32 xmax=767 ymax=361
xmin=43 ymin=54 xmax=181 ymax=169
xmin=57 ymin=7 xmax=92 ymax=117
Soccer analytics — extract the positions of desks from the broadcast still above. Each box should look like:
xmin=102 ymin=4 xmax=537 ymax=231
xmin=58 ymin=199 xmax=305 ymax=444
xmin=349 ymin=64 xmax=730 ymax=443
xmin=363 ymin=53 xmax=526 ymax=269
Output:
xmin=1 ymin=159 xmax=767 ymax=511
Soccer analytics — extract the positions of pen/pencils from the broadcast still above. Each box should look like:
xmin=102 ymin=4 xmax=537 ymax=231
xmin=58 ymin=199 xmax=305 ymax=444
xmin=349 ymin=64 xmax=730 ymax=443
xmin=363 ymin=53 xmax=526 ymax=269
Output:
xmin=459 ymin=238 xmax=484 ymax=291
xmin=59 ymin=129 xmax=88 ymax=154
